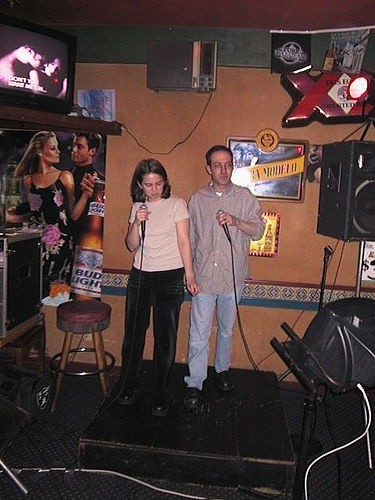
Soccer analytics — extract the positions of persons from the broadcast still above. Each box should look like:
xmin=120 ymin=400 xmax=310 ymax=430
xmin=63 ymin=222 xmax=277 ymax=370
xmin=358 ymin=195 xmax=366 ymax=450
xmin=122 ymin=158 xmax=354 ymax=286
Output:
xmin=6 ymin=131 xmax=100 ymax=299
xmin=182 ymin=145 xmax=265 ymax=406
xmin=118 ymin=158 xmax=201 ymax=414
xmin=0 ymin=36 xmax=67 ymax=98
xmin=7 ymin=131 xmax=105 ymax=239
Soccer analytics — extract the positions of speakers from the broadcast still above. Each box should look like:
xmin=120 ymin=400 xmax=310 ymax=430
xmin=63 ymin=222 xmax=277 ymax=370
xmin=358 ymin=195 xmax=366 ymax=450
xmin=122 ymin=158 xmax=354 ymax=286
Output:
xmin=317 ymin=139 xmax=375 ymax=241
xmin=301 ymin=296 xmax=375 ymax=393
xmin=147 ymin=40 xmax=217 ymax=90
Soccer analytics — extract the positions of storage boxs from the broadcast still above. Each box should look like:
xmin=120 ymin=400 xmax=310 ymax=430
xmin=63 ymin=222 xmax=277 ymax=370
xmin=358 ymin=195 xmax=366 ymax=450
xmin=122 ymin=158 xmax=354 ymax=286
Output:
xmin=0 ymin=229 xmax=43 ymax=339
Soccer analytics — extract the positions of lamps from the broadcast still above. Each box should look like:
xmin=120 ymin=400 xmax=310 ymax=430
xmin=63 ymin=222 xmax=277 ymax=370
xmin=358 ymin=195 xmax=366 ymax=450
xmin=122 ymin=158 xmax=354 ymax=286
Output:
xmin=346 ymin=72 xmax=374 ymax=105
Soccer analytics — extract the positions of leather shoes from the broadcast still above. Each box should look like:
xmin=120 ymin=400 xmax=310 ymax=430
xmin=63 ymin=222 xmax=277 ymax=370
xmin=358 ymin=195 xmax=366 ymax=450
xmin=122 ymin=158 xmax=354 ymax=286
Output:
xmin=182 ymin=388 xmax=200 ymax=408
xmin=217 ymin=369 xmax=233 ymax=391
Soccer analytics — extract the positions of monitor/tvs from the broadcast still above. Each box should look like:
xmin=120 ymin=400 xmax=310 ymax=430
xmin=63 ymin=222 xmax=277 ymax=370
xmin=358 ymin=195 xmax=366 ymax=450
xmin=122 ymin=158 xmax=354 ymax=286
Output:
xmin=0 ymin=14 xmax=77 ymax=117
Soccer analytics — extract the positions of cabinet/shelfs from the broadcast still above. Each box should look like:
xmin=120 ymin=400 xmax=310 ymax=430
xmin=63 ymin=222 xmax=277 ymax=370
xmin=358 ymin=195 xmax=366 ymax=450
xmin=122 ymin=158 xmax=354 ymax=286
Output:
xmin=0 ymin=105 xmax=122 ymax=351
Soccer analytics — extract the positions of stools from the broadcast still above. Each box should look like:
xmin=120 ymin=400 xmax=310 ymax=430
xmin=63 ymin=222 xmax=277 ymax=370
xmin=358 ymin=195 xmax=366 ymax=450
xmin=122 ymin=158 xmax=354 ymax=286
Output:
xmin=48 ymin=301 xmax=116 ymax=412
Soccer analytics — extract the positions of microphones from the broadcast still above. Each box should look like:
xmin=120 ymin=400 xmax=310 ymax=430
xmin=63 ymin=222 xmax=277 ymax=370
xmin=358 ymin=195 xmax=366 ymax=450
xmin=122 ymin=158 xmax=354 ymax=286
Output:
xmin=140 ymin=205 xmax=146 ymax=240
xmin=217 ymin=210 xmax=231 ymax=243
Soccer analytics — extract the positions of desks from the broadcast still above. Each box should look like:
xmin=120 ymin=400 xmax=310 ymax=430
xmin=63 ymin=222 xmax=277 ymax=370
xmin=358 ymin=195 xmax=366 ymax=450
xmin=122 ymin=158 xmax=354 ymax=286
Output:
xmin=0 ymin=312 xmax=47 ymax=397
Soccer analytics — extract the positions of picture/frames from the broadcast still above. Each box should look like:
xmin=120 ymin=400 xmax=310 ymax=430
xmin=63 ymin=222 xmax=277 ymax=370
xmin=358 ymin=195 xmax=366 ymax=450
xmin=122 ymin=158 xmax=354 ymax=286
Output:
xmin=225 ymin=137 xmax=309 ymax=203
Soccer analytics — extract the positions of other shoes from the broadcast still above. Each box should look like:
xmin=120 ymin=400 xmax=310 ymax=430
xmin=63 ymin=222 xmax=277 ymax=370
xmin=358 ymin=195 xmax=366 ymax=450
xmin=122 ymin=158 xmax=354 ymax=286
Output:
xmin=152 ymin=392 xmax=171 ymax=416
xmin=118 ymin=381 xmax=135 ymax=405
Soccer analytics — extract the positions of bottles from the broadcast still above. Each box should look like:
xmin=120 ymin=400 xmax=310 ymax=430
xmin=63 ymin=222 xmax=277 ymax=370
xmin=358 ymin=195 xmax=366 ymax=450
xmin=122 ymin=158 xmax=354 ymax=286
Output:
xmin=321 ymin=42 xmax=335 ymax=71
xmin=69 ymin=182 xmax=105 ymax=301
